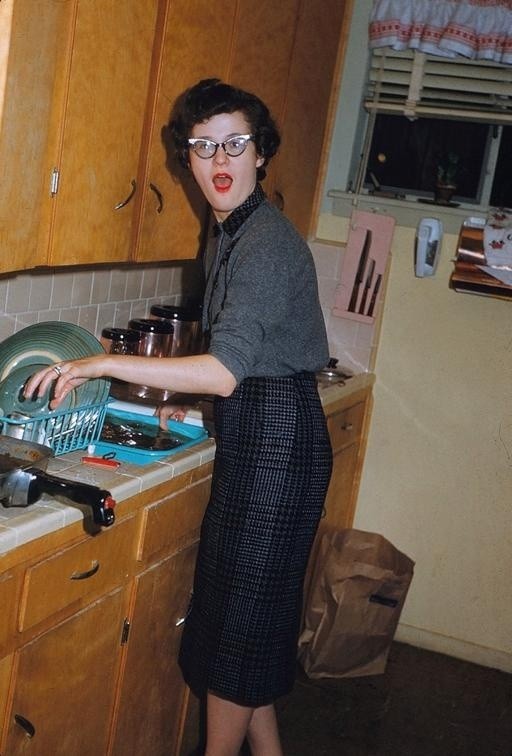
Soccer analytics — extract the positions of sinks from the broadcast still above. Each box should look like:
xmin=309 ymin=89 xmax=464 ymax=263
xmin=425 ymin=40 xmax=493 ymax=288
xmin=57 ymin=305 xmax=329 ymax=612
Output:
xmin=85 ymin=402 xmax=209 ymax=466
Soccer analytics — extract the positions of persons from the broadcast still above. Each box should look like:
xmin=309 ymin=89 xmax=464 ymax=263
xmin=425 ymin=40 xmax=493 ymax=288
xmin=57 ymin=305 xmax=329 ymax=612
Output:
xmin=22 ymin=77 xmax=333 ymax=756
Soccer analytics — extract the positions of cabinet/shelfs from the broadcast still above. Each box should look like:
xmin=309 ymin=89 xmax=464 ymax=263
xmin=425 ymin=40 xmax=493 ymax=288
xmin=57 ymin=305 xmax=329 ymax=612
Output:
xmin=0 ymin=0 xmax=76 ymax=273
xmin=0 ymin=513 xmax=135 ymax=754
xmin=46 ymin=2 xmax=236 ymax=274
xmin=110 ymin=475 xmax=217 ymax=753
xmin=235 ymin=0 xmax=354 ymax=243
xmin=322 ymin=406 xmax=365 ymax=531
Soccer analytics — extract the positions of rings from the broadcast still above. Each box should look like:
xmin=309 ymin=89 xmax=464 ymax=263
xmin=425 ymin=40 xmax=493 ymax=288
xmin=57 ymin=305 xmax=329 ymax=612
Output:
xmin=52 ymin=366 xmax=62 ymax=377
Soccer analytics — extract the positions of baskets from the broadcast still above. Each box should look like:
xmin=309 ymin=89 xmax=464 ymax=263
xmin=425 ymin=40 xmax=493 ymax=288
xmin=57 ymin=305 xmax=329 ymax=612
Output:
xmin=0 ymin=396 xmax=116 ymax=458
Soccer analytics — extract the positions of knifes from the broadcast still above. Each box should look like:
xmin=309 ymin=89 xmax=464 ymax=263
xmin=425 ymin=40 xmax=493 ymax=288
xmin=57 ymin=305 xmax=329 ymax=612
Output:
xmin=348 ymin=229 xmax=372 ymax=312
xmin=358 ymin=258 xmax=376 ymax=316
xmin=367 ymin=274 xmax=382 ymax=317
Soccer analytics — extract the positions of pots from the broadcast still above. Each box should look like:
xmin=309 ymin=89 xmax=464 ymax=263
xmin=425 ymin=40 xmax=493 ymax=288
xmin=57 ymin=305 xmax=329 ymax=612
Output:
xmin=315 ymin=355 xmax=358 ymax=389
xmin=0 ymin=434 xmax=117 ymax=528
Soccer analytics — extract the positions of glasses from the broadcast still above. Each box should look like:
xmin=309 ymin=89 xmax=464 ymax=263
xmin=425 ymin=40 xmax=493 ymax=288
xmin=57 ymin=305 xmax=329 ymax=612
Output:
xmin=188 ymin=134 xmax=255 ymax=159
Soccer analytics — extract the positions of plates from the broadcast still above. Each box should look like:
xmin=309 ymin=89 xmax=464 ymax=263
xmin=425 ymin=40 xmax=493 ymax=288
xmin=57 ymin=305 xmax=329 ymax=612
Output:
xmin=0 ymin=320 xmax=114 ymax=441
